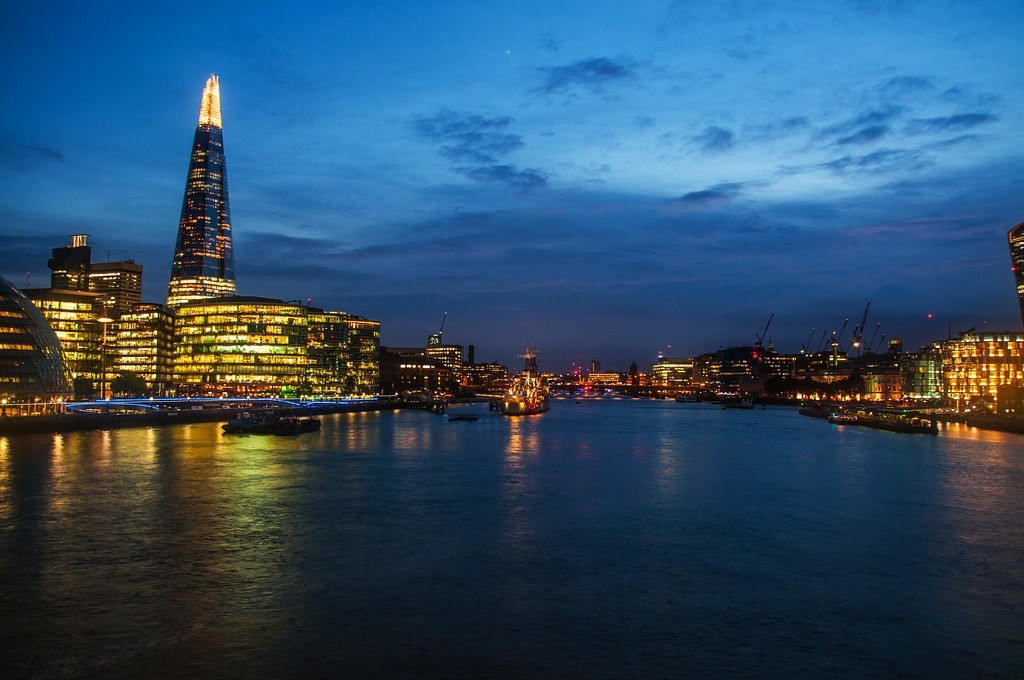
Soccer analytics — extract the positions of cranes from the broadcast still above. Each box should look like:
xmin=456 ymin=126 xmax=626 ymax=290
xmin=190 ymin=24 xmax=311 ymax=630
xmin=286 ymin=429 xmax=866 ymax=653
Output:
xmin=752 ymin=302 xmax=889 ymax=360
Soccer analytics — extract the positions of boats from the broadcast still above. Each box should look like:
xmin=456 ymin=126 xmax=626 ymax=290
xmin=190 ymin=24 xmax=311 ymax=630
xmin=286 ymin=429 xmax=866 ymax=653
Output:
xmin=496 ymin=339 xmax=551 ymax=415
xmin=221 ymin=403 xmax=322 ymax=436
